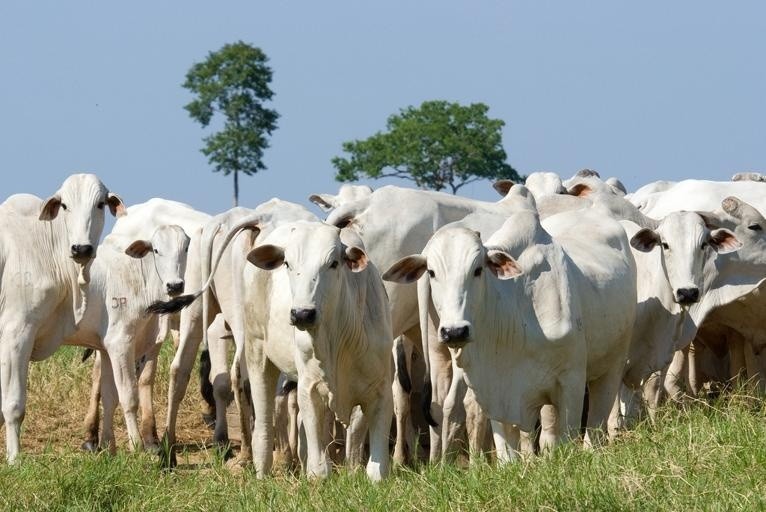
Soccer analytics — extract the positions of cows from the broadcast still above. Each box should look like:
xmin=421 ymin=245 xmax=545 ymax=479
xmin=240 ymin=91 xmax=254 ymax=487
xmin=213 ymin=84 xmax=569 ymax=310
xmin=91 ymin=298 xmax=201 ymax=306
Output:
xmin=1 ymin=169 xmax=765 ymax=488
